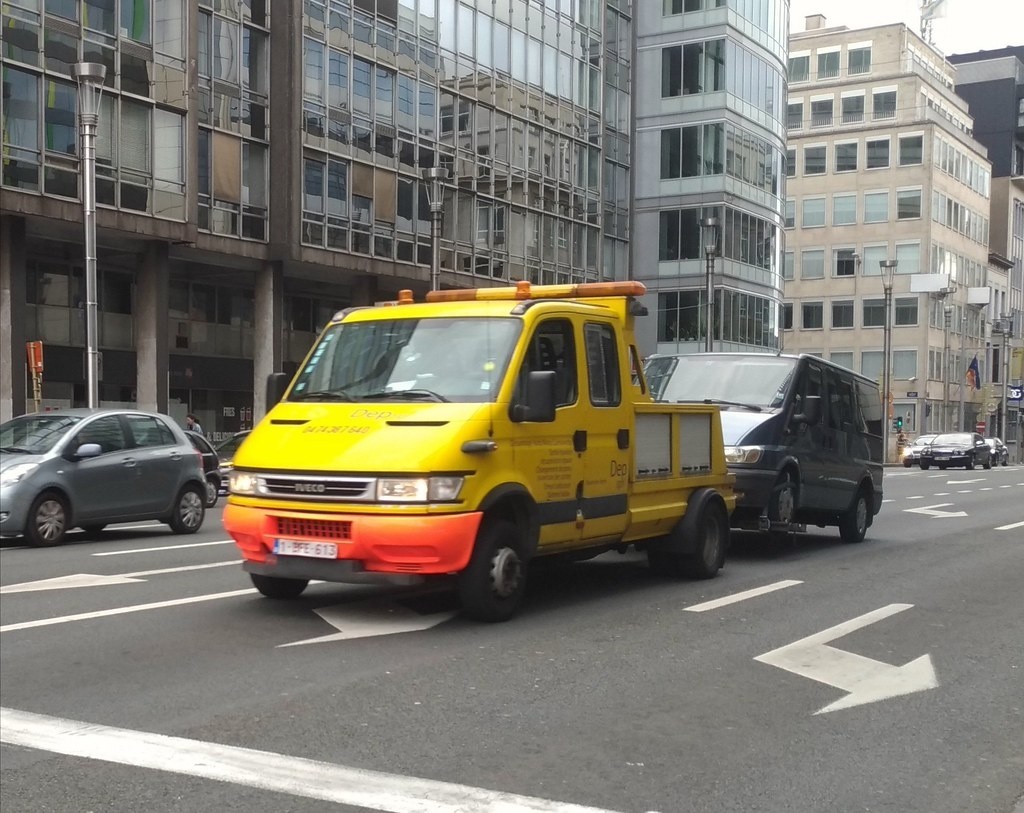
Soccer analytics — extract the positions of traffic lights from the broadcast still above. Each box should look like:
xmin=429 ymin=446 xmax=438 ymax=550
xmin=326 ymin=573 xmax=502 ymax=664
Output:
xmin=898 ymin=416 xmax=902 ymax=427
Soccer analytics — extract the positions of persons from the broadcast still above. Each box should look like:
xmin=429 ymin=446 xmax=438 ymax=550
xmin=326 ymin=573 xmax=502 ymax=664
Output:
xmin=186 ymin=415 xmax=204 ymax=436
xmin=896 ymin=428 xmax=908 ymax=463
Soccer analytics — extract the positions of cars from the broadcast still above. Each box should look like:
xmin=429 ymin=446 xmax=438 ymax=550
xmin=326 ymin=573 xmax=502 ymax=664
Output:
xmin=902 ymin=432 xmax=1009 ymax=470
xmin=0 ymin=407 xmax=253 ymax=547
xmin=221 ymin=281 xmax=736 ymax=624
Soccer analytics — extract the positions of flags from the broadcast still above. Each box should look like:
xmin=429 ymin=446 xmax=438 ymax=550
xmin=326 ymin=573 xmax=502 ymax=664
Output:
xmin=965 ymin=353 xmax=981 ymax=392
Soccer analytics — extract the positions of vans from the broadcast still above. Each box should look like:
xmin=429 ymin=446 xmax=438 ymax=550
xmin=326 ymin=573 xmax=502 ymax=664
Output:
xmin=634 ymin=346 xmax=884 ymax=542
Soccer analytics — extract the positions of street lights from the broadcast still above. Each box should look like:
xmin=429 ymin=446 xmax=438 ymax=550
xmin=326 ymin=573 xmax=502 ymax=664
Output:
xmin=69 ymin=62 xmax=106 ymax=408
xmin=879 ymin=260 xmax=898 ymax=463
xmin=1000 ymin=312 xmax=1014 ymax=446
xmin=940 ymin=287 xmax=957 ymax=433
xmin=699 ymin=216 xmax=720 ymax=352
xmin=422 ymin=167 xmax=449 ymax=290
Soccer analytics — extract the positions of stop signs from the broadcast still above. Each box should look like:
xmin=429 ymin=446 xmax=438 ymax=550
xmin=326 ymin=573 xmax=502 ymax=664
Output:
xmin=976 ymin=422 xmax=986 ymax=432
